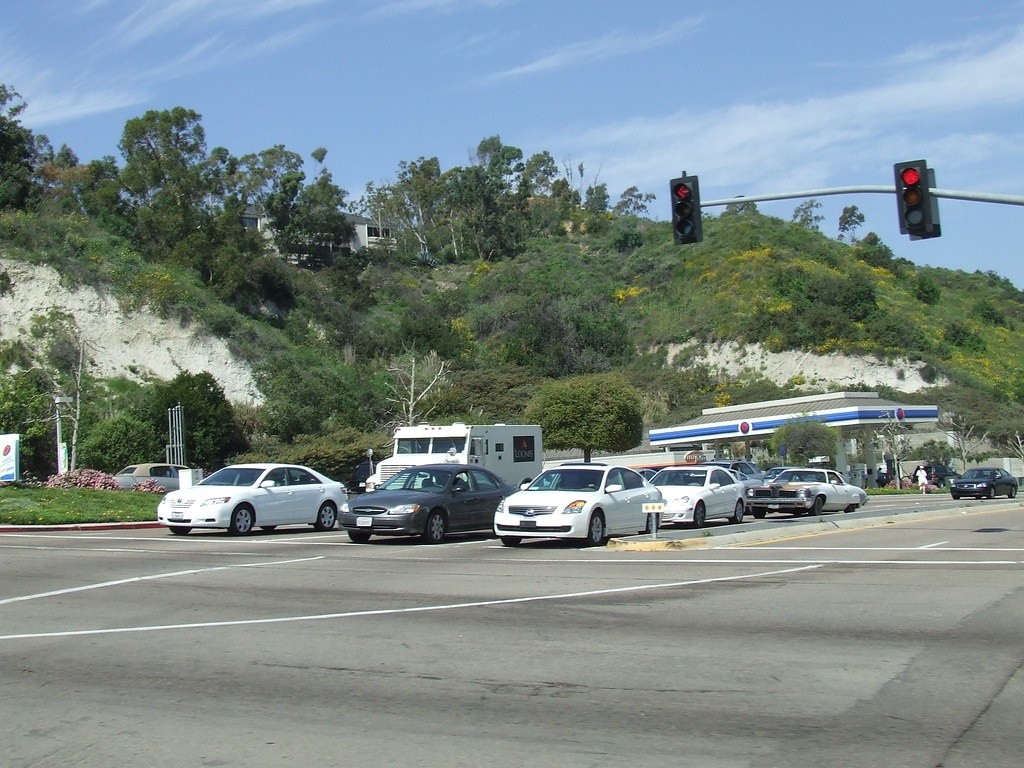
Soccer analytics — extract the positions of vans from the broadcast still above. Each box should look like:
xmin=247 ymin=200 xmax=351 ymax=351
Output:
xmin=364 ymin=421 xmax=543 ymax=496
xmin=157 ymin=462 xmax=348 ymax=535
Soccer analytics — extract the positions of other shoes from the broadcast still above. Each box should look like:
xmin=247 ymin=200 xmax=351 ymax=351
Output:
xmin=919 ymin=487 xmax=922 ymax=490
xmin=923 ymin=492 xmax=925 ymax=494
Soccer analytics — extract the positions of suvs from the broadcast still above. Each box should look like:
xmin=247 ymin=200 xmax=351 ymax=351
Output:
xmin=912 ymin=464 xmax=963 ymax=487
xmin=703 ymin=459 xmax=764 ymax=480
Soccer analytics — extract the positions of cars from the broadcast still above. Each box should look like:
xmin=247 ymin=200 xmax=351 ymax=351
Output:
xmin=336 ymin=463 xmax=519 ymax=545
xmin=493 ymin=463 xmax=664 ymax=547
xmin=744 ymin=469 xmax=869 ymax=517
xmin=727 ymin=468 xmax=764 ymax=515
xmin=631 ymin=467 xmax=658 ymax=483
xmin=645 ymin=465 xmax=748 ymax=528
xmin=111 ymin=463 xmax=191 ymax=491
xmin=761 ymin=466 xmax=802 ymax=486
xmin=950 ymin=467 xmax=1019 ymax=500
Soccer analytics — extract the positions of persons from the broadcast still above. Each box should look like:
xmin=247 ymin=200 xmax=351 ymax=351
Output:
xmin=915 ymin=466 xmax=928 ymax=494
xmin=877 ymin=467 xmax=882 ymax=487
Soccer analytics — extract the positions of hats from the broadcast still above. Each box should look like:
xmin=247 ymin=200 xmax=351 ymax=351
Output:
xmin=920 ymin=466 xmax=924 ymax=468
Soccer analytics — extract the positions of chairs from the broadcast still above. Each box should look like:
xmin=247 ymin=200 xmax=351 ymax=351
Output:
xmin=300 ymin=475 xmax=310 ymax=481
xmin=453 ymin=478 xmax=465 ymax=485
xmin=422 ymin=479 xmax=435 ymax=488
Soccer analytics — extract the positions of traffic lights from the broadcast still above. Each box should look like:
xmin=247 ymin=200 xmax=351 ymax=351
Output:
xmin=893 ymin=159 xmax=934 ymax=235
xmin=669 ymin=175 xmax=703 ymax=245
